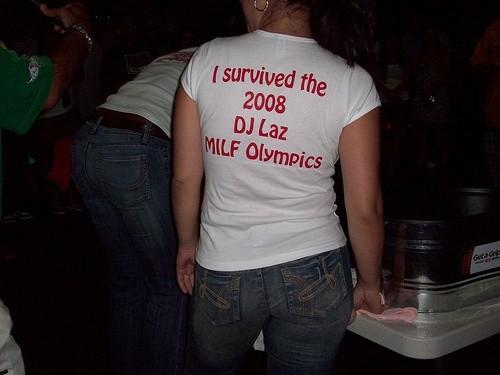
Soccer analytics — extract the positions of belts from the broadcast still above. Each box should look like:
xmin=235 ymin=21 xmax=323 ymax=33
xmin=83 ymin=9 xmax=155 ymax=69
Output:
xmin=87 ymin=113 xmax=169 ymax=139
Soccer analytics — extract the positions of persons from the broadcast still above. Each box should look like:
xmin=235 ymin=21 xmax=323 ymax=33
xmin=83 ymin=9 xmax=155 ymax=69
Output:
xmin=0 ymin=0 xmax=95 ymax=136
xmin=68 ymin=42 xmax=221 ymax=374
xmin=165 ymin=0 xmax=390 ymax=375
xmin=470 ymin=18 xmax=500 ymax=184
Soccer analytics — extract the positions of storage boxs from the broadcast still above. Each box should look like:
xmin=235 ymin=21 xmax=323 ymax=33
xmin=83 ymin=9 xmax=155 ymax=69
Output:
xmin=343 ymin=202 xmax=500 ymax=314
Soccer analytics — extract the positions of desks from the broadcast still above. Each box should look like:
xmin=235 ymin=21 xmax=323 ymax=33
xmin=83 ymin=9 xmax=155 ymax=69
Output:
xmin=328 ymin=295 xmax=500 ymax=375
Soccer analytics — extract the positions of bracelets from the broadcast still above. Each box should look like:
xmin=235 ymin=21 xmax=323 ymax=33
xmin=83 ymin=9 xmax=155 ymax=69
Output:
xmin=71 ymin=23 xmax=94 ymax=51
